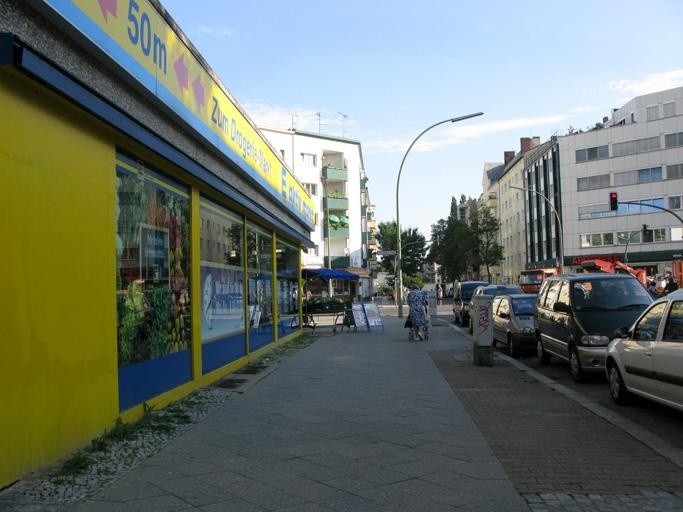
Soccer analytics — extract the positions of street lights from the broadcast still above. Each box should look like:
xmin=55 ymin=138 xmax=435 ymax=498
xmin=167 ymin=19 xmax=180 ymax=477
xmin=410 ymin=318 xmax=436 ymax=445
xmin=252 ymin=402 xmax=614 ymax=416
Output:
xmin=625 ymin=223 xmax=653 ymax=263
xmin=321 ymin=176 xmax=333 ymax=300
xmin=395 ymin=111 xmax=485 ymax=318
xmin=509 ymin=185 xmax=565 ymax=272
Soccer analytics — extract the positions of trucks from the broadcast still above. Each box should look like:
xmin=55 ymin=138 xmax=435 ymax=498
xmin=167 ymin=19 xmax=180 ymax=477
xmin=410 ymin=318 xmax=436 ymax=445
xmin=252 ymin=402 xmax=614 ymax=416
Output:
xmin=519 ymin=269 xmax=558 ymax=294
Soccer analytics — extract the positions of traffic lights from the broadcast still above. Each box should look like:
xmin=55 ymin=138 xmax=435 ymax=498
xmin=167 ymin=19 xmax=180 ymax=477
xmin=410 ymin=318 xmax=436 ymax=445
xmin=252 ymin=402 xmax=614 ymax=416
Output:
xmin=610 ymin=192 xmax=618 ymax=211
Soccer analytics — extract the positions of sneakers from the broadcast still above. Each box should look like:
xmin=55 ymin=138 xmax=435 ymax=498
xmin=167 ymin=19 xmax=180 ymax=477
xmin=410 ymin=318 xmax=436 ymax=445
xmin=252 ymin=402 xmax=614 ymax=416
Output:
xmin=415 ymin=332 xmax=424 ymax=341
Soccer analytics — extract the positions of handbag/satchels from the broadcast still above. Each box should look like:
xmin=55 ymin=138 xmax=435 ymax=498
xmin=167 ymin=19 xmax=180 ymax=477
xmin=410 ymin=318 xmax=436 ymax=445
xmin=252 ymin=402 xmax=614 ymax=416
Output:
xmin=404 ymin=319 xmax=413 ymax=329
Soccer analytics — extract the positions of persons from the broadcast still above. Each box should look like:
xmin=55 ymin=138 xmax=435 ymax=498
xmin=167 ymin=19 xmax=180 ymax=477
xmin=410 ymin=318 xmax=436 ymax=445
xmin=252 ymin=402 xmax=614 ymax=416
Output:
xmin=661 ymin=278 xmax=678 ymax=296
xmin=404 ymin=284 xmax=428 ymax=341
xmin=437 ymin=284 xmax=443 ymax=306
xmin=647 ymin=282 xmax=662 ymax=294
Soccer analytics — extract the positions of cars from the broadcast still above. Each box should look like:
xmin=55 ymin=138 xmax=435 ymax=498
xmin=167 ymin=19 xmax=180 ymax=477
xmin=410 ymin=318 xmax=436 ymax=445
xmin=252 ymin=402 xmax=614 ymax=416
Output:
xmin=453 ymin=273 xmax=683 ymax=410
